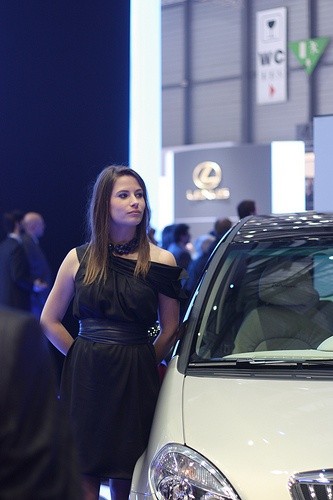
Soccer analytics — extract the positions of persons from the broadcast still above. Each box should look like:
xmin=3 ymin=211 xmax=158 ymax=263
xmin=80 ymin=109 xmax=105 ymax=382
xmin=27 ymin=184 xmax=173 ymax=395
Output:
xmin=39 ymin=165 xmax=188 ymax=500
xmin=0 ymin=210 xmax=61 ymax=318
xmin=162 ymin=199 xmax=255 ymax=298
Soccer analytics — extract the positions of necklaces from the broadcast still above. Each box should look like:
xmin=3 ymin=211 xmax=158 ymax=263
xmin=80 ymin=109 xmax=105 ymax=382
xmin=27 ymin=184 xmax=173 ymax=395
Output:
xmin=108 ymin=233 xmax=140 ymax=255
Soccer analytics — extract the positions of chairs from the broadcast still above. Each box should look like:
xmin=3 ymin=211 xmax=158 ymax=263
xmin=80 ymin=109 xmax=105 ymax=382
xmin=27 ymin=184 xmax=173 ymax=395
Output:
xmin=232 ymin=262 xmax=331 ymax=354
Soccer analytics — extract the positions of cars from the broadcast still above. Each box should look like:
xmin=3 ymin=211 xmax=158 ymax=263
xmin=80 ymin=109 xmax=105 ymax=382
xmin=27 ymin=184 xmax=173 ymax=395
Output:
xmin=124 ymin=204 xmax=333 ymax=500
